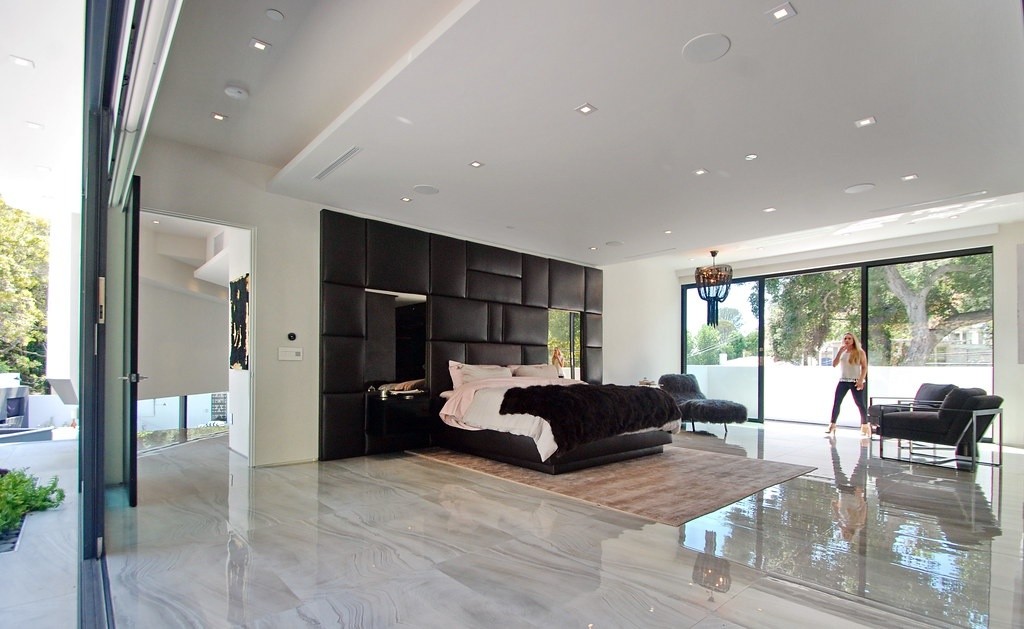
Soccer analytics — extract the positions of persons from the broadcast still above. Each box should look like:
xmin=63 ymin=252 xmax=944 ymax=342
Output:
xmin=825 ymin=429 xmax=869 ymax=544
xmin=552 ymin=347 xmax=565 ymax=378
xmin=825 ymin=333 xmax=872 ymax=437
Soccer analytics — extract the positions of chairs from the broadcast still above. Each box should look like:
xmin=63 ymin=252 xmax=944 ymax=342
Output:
xmin=868 ymin=384 xmax=959 ymax=449
xmin=659 ymin=374 xmax=748 ymax=433
xmin=879 ymin=388 xmax=1003 ymax=473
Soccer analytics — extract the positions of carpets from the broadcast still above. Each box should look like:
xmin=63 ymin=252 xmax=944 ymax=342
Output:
xmin=403 ymin=444 xmax=819 ymax=528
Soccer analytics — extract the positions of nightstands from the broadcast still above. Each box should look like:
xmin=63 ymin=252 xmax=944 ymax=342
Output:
xmin=364 ymin=388 xmax=431 ymax=454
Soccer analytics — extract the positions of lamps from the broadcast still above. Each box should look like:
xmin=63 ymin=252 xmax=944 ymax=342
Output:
xmin=691 ymin=531 xmax=731 ymax=604
xmin=695 ymin=250 xmax=733 ymax=328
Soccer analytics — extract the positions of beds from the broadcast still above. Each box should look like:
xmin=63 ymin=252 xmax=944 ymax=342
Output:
xmin=435 ymin=360 xmax=682 ymax=476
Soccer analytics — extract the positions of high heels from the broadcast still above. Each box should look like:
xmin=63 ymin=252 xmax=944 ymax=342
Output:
xmin=825 ymin=423 xmax=836 ymax=434
xmin=824 ymin=434 xmax=836 ymax=447
xmin=861 ymin=423 xmax=871 ymax=437
xmin=860 ymin=438 xmax=871 ymax=447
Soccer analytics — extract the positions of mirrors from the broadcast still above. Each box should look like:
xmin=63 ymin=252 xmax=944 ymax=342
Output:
xmin=548 ymin=309 xmax=581 ymax=380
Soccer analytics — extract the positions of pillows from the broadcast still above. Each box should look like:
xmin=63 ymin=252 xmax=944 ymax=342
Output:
xmin=448 ymin=360 xmax=501 ymax=388
xmin=514 ymin=365 xmax=558 ymax=378
xmin=460 ymin=365 xmax=512 ymax=386
xmin=507 ymin=363 xmax=547 ymax=376
